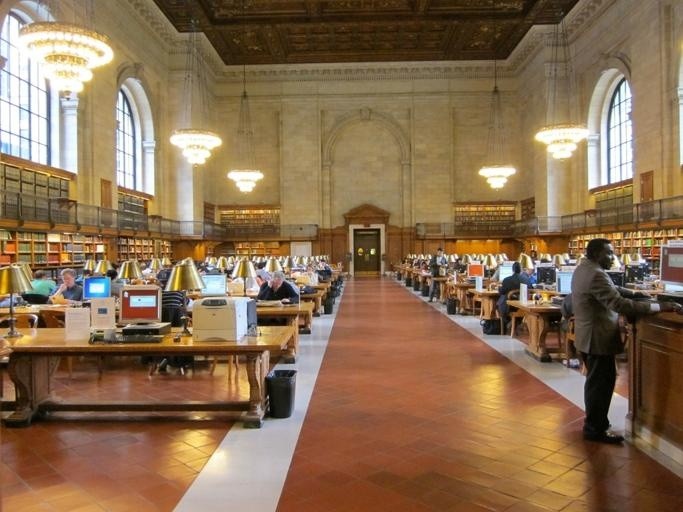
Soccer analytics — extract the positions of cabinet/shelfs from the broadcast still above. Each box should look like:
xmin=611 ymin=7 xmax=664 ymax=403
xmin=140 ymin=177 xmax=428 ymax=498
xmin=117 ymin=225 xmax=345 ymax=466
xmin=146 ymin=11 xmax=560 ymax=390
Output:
xmin=566 ymin=225 xmax=683 ymax=266
xmin=0 ymin=226 xmax=172 ymax=284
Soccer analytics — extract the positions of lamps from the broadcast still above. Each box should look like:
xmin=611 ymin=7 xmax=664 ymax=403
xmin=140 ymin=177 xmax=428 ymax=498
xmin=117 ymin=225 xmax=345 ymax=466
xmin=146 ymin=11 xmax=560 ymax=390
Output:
xmin=170 ymin=0 xmax=222 ymax=166
xmin=405 ymin=252 xmax=633 ymax=284
xmin=535 ymin=0 xmax=590 ymax=161
xmin=17 ymin=0 xmax=115 ymax=100
xmin=478 ymin=0 xmax=515 ymax=191
xmin=227 ymin=0 xmax=264 ymax=193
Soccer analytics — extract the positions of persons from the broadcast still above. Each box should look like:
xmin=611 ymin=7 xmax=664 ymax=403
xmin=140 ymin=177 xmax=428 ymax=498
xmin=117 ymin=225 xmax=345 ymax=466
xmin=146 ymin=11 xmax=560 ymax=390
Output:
xmin=26 ymin=260 xmax=340 ymax=324
xmin=561 ymin=293 xmax=580 ymax=369
xmin=402 ymin=248 xmax=503 ymax=302
xmin=496 ymin=261 xmax=532 ymax=336
xmin=571 ymin=238 xmax=676 ymax=443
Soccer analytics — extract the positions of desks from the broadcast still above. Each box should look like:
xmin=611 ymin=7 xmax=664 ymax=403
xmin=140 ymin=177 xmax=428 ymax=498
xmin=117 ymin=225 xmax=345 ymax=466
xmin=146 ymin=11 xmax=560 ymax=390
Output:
xmin=392 ymin=262 xmax=661 ymax=363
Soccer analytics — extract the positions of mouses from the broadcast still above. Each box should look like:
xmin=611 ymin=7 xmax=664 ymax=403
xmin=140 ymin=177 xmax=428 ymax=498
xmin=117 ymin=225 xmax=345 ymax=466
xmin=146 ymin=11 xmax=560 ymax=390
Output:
xmin=173 ymin=336 xmax=179 ymax=341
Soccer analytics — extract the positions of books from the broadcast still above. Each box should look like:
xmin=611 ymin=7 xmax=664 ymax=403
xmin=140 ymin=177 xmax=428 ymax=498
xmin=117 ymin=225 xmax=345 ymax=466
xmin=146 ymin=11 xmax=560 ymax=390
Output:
xmin=569 ymin=228 xmax=683 ymax=256
xmin=0 ymin=231 xmax=173 ymax=278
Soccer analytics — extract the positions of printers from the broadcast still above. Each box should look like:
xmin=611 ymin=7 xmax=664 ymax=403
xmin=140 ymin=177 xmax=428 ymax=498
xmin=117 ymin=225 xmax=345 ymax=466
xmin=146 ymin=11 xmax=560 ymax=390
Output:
xmin=192 ymin=296 xmax=248 ymax=341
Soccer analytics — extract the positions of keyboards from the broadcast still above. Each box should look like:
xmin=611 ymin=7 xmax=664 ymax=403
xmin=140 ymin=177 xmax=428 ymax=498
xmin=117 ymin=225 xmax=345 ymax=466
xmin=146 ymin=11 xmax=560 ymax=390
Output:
xmin=93 ymin=333 xmax=163 ymax=343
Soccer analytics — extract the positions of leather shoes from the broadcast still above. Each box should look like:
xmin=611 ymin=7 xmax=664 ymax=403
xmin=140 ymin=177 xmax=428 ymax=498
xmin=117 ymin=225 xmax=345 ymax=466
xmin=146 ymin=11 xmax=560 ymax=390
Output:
xmin=585 ymin=430 xmax=625 ymax=445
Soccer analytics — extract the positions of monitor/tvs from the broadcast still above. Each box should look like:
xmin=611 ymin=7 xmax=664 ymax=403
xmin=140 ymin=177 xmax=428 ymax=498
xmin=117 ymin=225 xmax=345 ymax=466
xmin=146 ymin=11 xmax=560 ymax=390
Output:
xmin=118 ymin=287 xmax=162 ymax=323
xmin=659 ymin=245 xmax=683 ymax=286
xmin=201 ymin=274 xmax=225 ymax=296
xmin=468 ymin=261 xmax=646 ymax=295
xmin=82 ymin=276 xmax=112 ymax=300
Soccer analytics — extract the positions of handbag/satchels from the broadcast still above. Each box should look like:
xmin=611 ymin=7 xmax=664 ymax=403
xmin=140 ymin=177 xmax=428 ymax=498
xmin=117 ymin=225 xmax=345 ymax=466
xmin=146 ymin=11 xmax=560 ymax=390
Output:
xmin=478 ymin=318 xmax=507 ymax=335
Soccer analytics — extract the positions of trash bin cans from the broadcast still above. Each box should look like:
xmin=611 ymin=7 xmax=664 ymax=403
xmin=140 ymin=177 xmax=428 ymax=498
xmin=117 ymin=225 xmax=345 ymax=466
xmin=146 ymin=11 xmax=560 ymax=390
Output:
xmin=397 ymin=273 xmax=401 ymax=280
xmin=414 ymin=281 xmax=420 ymax=290
xmin=447 ymin=300 xmax=456 ymax=314
xmin=423 ymin=286 xmax=429 ymax=296
xmin=406 ymin=277 xmax=411 ymax=286
xmin=324 ymin=276 xmax=343 ymax=314
xmin=266 ymin=370 xmax=297 ymax=419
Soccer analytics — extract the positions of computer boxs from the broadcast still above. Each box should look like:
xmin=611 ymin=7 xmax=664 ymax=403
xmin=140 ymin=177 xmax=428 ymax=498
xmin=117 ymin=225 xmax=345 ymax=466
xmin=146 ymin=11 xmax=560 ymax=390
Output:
xmin=121 ymin=321 xmax=171 ymax=335
xmin=657 ymin=292 xmax=683 ymax=312
